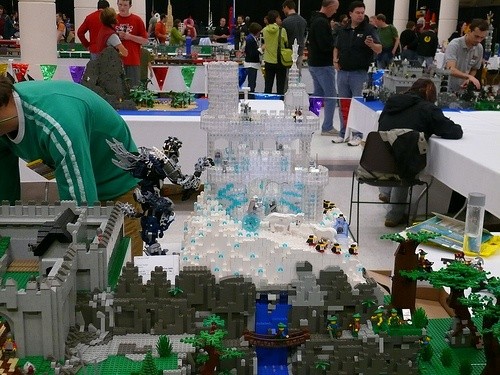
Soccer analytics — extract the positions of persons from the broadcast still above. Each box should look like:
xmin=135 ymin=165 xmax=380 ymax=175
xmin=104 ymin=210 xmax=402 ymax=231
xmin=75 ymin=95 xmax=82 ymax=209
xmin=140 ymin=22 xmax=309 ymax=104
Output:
xmin=0 ymin=0 xmax=500 ymax=147
xmin=356 ymin=78 xmax=463 ymax=230
xmin=0 ymin=80 xmax=143 ymax=238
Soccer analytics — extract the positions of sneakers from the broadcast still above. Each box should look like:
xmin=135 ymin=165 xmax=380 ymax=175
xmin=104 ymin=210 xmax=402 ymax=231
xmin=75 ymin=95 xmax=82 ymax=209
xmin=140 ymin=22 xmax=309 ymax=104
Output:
xmin=331 ymin=136 xmax=347 ymax=143
xmin=321 ymin=127 xmax=340 ymax=136
xmin=347 ymin=137 xmax=364 ymax=146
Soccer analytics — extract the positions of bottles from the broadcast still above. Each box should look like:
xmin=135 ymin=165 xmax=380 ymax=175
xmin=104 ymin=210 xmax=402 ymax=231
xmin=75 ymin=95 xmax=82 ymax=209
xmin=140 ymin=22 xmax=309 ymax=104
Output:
xmin=463 ymin=192 xmax=486 ymax=252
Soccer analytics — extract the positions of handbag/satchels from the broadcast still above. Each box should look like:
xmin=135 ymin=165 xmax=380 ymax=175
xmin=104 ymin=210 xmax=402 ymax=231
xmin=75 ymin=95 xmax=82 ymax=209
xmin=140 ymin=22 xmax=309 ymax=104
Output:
xmin=392 ymin=42 xmax=400 ymax=56
xmin=280 ymin=48 xmax=293 ymax=66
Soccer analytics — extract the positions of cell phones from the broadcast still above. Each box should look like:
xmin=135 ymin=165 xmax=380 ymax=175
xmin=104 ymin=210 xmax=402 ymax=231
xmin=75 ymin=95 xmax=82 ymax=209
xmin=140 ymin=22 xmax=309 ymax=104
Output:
xmin=367 ymin=36 xmax=372 ymax=39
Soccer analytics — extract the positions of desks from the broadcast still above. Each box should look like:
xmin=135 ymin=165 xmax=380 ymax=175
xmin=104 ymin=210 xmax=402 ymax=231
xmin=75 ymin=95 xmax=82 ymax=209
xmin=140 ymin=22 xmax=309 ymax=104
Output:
xmin=344 ymin=97 xmax=500 ymax=218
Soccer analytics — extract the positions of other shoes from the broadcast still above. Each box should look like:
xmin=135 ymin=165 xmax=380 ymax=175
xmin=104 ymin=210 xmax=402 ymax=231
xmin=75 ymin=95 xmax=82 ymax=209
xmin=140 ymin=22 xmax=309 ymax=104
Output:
xmin=375 ymin=184 xmax=409 ymax=228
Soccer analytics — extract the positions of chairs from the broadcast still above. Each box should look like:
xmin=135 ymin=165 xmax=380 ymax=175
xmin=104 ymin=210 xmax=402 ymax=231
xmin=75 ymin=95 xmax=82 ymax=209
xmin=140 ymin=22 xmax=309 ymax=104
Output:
xmin=349 ymin=128 xmax=429 ymax=247
